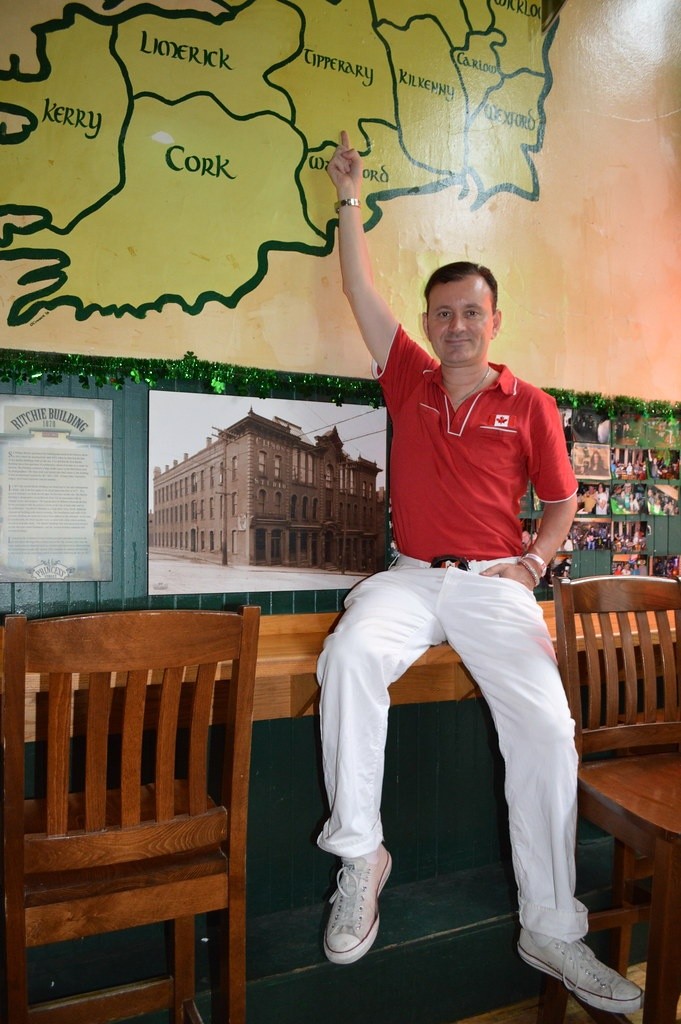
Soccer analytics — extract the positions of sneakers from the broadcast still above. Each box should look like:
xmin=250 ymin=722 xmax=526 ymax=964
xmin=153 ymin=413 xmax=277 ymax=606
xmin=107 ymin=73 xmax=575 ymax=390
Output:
xmin=517 ymin=925 xmax=644 ymax=1014
xmin=324 ymin=842 xmax=392 ymax=964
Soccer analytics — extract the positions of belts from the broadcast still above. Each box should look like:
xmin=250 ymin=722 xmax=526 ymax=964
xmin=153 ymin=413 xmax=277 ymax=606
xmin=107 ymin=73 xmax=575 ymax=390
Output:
xmin=396 ymin=551 xmax=521 ymax=573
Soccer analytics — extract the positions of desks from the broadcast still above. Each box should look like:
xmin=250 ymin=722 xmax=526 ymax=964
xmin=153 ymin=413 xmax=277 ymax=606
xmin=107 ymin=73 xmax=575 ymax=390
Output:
xmin=0 ymin=600 xmax=681 ymax=742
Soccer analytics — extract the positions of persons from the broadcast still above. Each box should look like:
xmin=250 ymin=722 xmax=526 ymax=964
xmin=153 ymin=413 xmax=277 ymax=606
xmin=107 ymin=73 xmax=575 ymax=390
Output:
xmin=522 ymin=409 xmax=681 ymax=580
xmin=314 ymin=130 xmax=644 ymax=1003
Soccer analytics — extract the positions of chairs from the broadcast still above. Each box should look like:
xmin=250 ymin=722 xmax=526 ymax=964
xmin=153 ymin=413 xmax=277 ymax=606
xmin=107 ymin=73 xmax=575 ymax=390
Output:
xmin=537 ymin=575 xmax=681 ymax=1024
xmin=0 ymin=604 xmax=261 ymax=1024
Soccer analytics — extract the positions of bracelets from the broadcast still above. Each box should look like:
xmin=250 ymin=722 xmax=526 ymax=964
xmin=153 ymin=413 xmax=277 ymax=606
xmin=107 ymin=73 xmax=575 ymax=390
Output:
xmin=333 ymin=198 xmax=362 ymax=213
xmin=523 ymin=553 xmax=546 ymax=577
xmin=517 ymin=559 xmax=539 ymax=587
